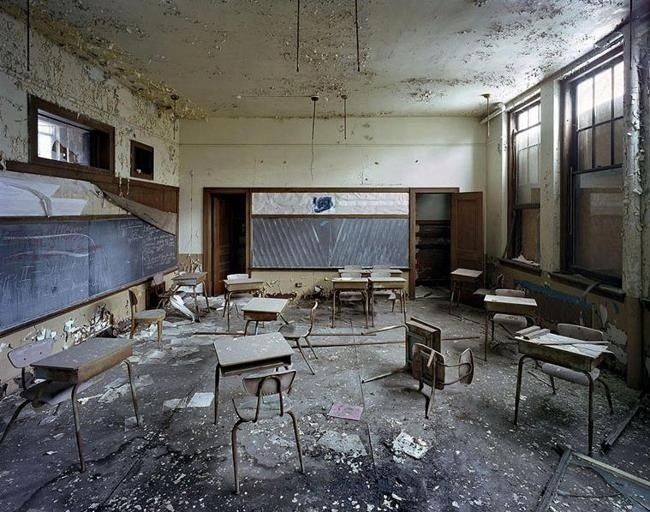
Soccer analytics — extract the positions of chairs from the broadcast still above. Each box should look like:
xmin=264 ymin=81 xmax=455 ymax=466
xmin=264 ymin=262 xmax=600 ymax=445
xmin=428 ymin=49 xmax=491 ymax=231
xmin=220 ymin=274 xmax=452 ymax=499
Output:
xmin=128 ymin=290 xmax=166 ymax=345
xmin=146 ymin=281 xmax=186 ymax=329
xmin=212 ymin=273 xmax=319 ymax=494
xmin=372 ymin=264 xmax=390 ymax=269
xmin=344 ymin=265 xmax=363 ymax=269
xmin=409 ymin=342 xmax=474 ymax=419
xmin=471 ymin=274 xmax=617 ymax=458
xmin=0 ymin=338 xmax=93 ymax=452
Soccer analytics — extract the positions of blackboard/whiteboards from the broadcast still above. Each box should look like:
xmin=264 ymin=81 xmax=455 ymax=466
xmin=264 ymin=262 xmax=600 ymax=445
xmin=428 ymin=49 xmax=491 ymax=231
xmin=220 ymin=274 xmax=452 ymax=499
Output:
xmin=249 ymin=214 xmax=411 ymax=272
xmin=0 ymin=215 xmax=180 ymax=339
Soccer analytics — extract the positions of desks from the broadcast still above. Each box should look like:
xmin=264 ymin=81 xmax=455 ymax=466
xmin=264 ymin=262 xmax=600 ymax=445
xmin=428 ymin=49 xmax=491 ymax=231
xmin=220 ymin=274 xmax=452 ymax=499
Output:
xmin=448 ymin=268 xmax=484 ymax=315
xmin=361 ymin=316 xmax=441 ymax=383
xmin=330 ymin=269 xmax=408 ymax=328
xmin=28 ymin=336 xmax=142 ymax=473
xmin=169 ymin=272 xmax=210 ymax=323
